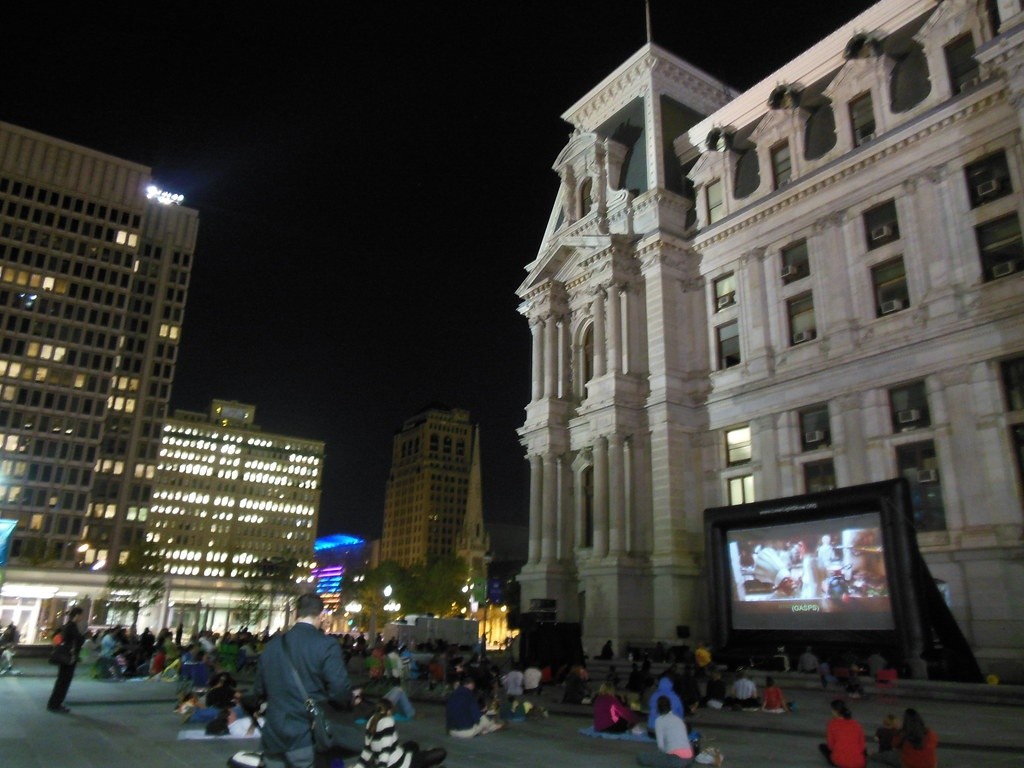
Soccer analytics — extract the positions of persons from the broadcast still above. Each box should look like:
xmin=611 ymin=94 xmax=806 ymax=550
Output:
xmin=258 ymin=592 xmax=354 ymax=768
xmin=356 ymin=699 xmax=447 ymax=768
xmin=0 ymin=620 xmax=887 ymax=766
xmin=818 ymin=698 xmax=867 ymax=768
xmin=47 ymin=607 xmax=92 ymax=714
xmin=874 ymin=707 xmax=939 ymax=768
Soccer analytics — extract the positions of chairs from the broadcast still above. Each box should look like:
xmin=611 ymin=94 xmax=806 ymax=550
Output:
xmin=378 ymin=655 xmax=394 ymax=695
xmin=218 ymin=643 xmax=228 ymax=672
xmin=224 ymin=644 xmax=250 ymax=681
xmin=364 ymin=656 xmax=382 ymax=694
xmin=177 ymin=662 xmax=209 ymax=700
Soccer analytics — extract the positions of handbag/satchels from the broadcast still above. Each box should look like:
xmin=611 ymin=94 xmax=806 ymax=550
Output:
xmin=308 ymin=698 xmax=363 ymax=758
xmin=49 ymin=645 xmax=72 ymax=666
xmin=697 ymin=747 xmax=723 ymax=767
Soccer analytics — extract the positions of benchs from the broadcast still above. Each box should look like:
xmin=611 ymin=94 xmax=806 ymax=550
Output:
xmin=739 ymin=669 xmax=1024 ymax=706
xmin=585 ymin=659 xmax=728 ymax=681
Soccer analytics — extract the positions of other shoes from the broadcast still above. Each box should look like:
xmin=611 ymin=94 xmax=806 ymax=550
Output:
xmin=482 ymin=723 xmax=503 ymax=734
xmin=413 ymin=712 xmax=425 ymax=721
xmin=47 ymin=704 xmax=68 ymax=715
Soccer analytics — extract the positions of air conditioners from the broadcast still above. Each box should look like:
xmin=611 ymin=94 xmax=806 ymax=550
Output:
xmin=781 ymin=265 xmax=795 ymax=278
xmin=992 ymin=261 xmax=1015 ymax=278
xmin=881 ymin=299 xmax=902 ymax=314
xmin=718 ymin=295 xmax=732 ymax=307
xmin=960 ymin=77 xmax=978 ymax=91
xmin=859 ymin=133 xmax=875 ymax=145
xmin=977 ymin=180 xmax=1000 ymax=197
xmin=793 ymin=329 xmax=812 ymax=344
xmin=779 ymin=178 xmax=790 ymax=188
xmin=898 ymin=409 xmax=920 ymax=423
xmin=917 ymin=469 xmax=937 ymax=483
xmin=806 ymin=430 xmax=824 ymax=443
xmin=872 ymin=225 xmax=892 ymax=241
